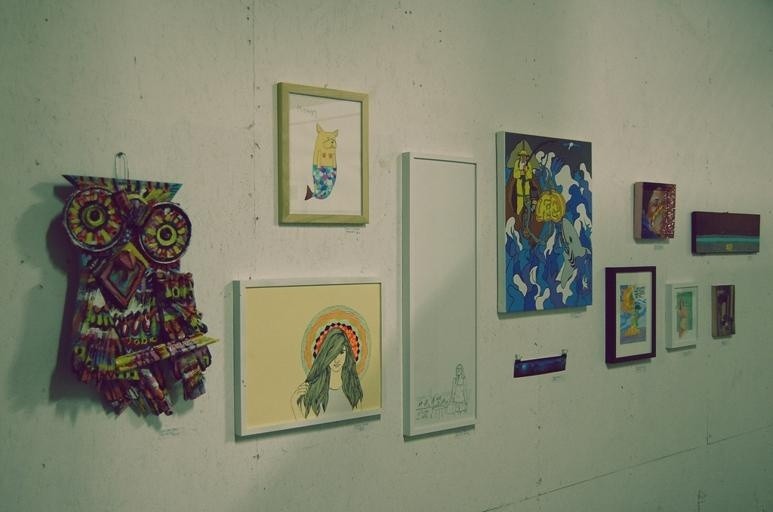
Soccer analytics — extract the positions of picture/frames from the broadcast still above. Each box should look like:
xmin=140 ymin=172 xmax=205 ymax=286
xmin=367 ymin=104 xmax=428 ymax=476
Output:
xmin=605 ymin=266 xmax=656 ymax=362
xmin=235 ymin=278 xmax=384 ymax=439
xmin=667 ymin=283 xmax=698 ymax=349
xmin=279 ymin=82 xmax=371 ymax=225
xmin=402 ymin=152 xmax=480 ymax=437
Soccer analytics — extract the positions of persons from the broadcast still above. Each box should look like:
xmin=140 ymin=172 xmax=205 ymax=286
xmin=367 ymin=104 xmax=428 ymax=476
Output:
xmin=289 ymin=328 xmax=363 ymax=423
xmin=677 ymin=297 xmax=687 ymax=338
xmin=511 ymin=150 xmax=533 ymax=215
xmin=448 ymin=363 xmax=468 ymax=419
